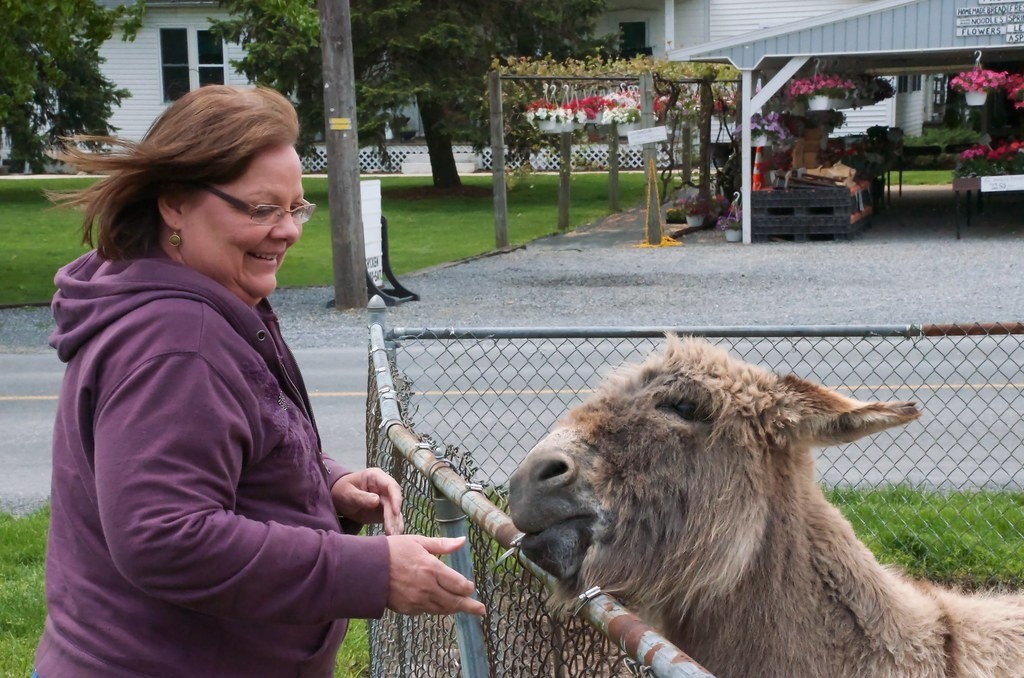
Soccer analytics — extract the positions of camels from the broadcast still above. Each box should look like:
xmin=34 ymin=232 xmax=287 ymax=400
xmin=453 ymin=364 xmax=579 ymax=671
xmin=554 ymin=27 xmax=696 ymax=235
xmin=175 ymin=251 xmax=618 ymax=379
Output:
xmin=508 ymin=332 xmax=1024 ymax=678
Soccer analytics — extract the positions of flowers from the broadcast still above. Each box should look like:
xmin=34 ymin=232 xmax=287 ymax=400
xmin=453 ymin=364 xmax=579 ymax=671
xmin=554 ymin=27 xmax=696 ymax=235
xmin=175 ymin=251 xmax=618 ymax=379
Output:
xmin=949 ymin=67 xmax=1007 ymax=91
xmin=717 ymin=206 xmax=743 ymax=230
xmin=681 ymin=195 xmax=706 ymax=216
xmin=787 ymin=72 xmax=895 ymax=99
xmin=951 ymin=142 xmax=1024 ymax=178
xmin=736 ymin=111 xmax=867 ymax=164
xmin=524 ymin=86 xmax=735 ymax=117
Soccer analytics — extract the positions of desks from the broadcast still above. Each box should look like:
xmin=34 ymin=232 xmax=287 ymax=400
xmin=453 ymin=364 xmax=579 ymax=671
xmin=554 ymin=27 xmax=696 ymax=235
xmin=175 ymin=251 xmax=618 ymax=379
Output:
xmin=954 ymin=175 xmax=1024 ymax=239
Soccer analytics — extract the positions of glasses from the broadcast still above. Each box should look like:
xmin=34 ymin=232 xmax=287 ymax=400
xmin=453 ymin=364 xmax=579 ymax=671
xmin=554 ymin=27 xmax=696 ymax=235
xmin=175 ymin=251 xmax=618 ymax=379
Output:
xmin=200 ymin=184 xmax=317 ymax=226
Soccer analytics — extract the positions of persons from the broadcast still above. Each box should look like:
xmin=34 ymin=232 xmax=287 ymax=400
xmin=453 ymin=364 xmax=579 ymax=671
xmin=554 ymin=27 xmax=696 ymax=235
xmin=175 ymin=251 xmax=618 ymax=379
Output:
xmin=32 ymin=85 xmax=488 ymax=678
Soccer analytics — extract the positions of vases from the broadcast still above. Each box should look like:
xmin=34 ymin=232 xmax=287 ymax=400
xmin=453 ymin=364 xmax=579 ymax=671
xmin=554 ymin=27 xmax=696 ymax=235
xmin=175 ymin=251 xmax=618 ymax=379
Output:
xmin=545 ymin=123 xmax=561 ymax=134
xmin=573 ymin=118 xmax=583 ymax=128
xmin=538 ymin=116 xmax=555 ymax=130
xmin=586 ymin=112 xmax=601 ymax=123
xmin=751 ymin=134 xmax=771 ymax=147
xmin=809 ymin=94 xmax=877 ymax=110
xmin=560 ymin=119 xmax=574 ymax=131
xmin=726 ymin=229 xmax=742 ymax=242
xmin=687 ymin=217 xmax=704 ymax=227
xmin=981 ymin=175 xmax=1024 ymax=191
xmin=964 ymin=89 xmax=987 ymax=105
xmin=820 ymin=127 xmax=828 ymax=149
